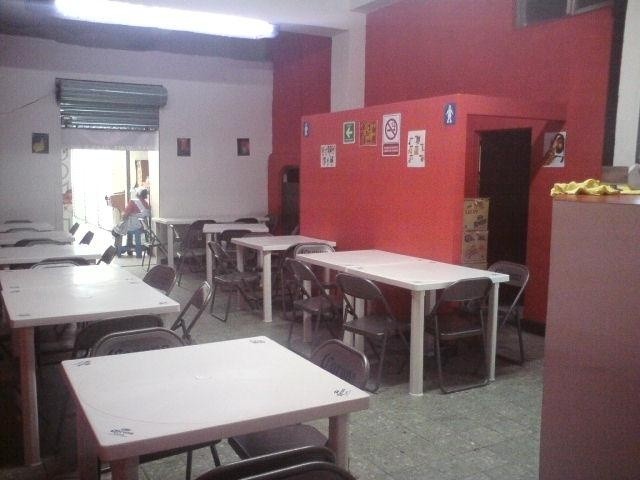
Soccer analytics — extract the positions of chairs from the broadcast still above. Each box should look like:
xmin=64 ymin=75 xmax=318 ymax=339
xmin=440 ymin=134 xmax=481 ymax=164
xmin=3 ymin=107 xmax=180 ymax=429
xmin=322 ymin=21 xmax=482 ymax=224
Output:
xmin=226 ymin=336 xmax=372 ymax=461
xmin=463 ymin=259 xmax=529 ymax=368
xmin=2 ymin=215 xmax=337 ymax=324
xmin=278 ymin=254 xmax=342 ymax=347
xmin=84 ymin=326 xmax=223 ymax=478
xmin=170 ymin=279 xmax=215 ymax=342
xmin=332 ymin=271 xmax=420 ymax=393
xmin=417 ymin=274 xmax=494 ymax=394
xmin=190 ymin=443 xmax=357 ymax=479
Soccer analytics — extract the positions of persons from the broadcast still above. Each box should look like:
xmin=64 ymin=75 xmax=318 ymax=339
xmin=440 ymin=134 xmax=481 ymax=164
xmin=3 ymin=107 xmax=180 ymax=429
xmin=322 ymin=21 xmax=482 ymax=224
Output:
xmin=113 ymin=221 xmax=127 ymax=258
xmin=122 ymin=189 xmax=150 ymax=258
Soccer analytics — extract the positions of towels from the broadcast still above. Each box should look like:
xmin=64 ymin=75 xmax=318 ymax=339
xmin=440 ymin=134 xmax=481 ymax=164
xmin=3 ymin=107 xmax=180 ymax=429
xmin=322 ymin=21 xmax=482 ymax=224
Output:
xmin=549 ymin=179 xmax=640 ymax=196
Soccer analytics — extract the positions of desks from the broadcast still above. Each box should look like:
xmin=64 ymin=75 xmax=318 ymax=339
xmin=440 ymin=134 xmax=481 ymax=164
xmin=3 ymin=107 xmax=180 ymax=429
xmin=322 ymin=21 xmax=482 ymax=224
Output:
xmin=57 ymin=333 xmax=376 ymax=478
xmin=3 ymin=261 xmax=182 ymax=469
xmin=294 ymin=245 xmax=511 ymax=398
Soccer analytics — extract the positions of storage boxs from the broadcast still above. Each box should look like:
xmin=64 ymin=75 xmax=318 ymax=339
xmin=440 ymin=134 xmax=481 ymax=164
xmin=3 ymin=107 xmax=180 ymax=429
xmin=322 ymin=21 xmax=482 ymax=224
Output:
xmin=462 ymin=197 xmax=488 ymax=269
